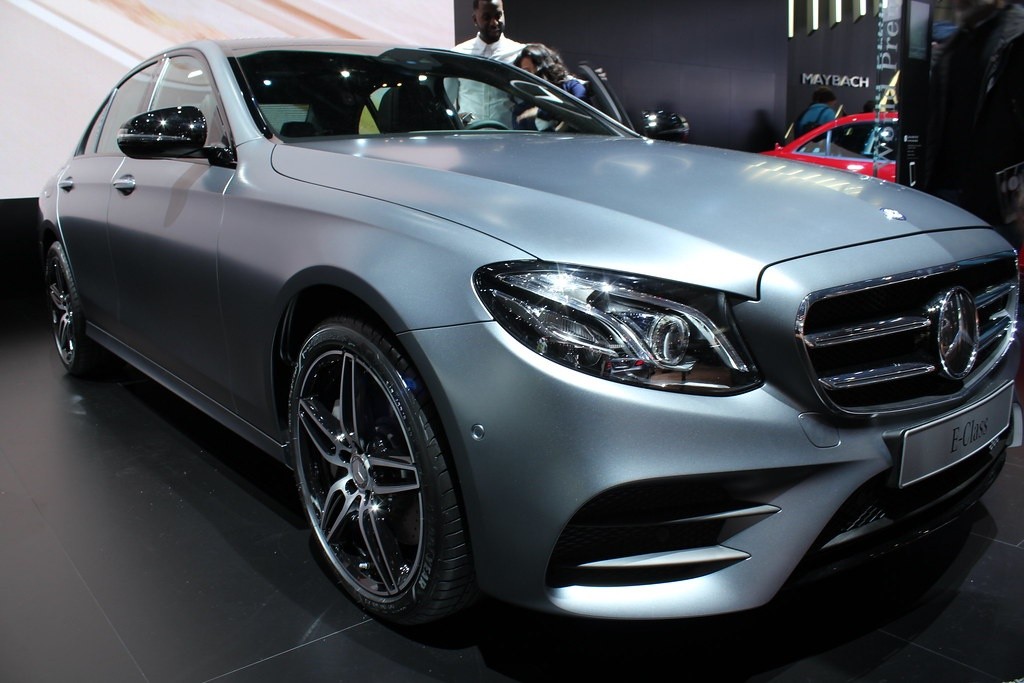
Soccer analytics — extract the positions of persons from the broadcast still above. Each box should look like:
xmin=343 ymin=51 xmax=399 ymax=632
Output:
xmin=855 ymin=99 xmax=875 ymax=152
xmin=511 ymin=45 xmax=608 ymax=132
xmin=444 ymin=0 xmax=526 ymax=130
xmin=924 ymin=0 xmax=1024 ymax=251
xmin=794 ymin=86 xmax=836 ymax=138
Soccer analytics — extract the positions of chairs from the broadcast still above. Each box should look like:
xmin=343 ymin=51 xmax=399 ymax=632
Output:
xmin=376 ymin=85 xmax=455 ymax=132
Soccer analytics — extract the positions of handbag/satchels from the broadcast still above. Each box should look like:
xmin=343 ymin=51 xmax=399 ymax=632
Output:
xmin=802 ymin=107 xmax=834 ymax=144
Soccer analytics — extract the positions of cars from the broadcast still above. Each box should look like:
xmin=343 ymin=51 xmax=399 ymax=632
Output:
xmin=36 ymin=37 xmax=1022 ymax=630
xmin=763 ymin=109 xmax=898 ymax=182
